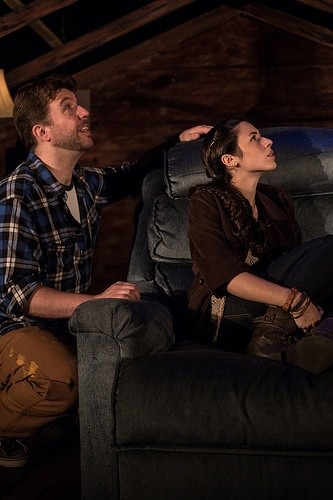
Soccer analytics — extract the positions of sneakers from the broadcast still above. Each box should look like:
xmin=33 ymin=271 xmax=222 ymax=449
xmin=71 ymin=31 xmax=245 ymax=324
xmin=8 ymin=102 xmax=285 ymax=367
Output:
xmin=0 ymin=436 xmax=30 ymax=468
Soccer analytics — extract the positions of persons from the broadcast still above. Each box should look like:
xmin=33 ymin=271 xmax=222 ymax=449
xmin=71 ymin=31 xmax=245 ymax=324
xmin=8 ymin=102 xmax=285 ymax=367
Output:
xmin=188 ymin=119 xmax=333 ymax=374
xmin=0 ymin=77 xmax=215 ymax=469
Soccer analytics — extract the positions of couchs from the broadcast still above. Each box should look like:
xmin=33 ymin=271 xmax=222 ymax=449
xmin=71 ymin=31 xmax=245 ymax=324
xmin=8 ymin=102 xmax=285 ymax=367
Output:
xmin=67 ymin=125 xmax=333 ymax=500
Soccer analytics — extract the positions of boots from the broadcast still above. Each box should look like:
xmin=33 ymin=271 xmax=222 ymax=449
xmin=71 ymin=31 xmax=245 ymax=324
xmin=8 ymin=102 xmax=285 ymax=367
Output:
xmin=286 ymin=317 xmax=333 ymax=374
xmin=246 ymin=304 xmax=298 ymax=362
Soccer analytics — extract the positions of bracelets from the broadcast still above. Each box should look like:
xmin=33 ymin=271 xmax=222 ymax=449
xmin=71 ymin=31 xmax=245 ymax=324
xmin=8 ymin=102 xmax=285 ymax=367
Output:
xmin=282 ymin=288 xmax=310 ymax=318
